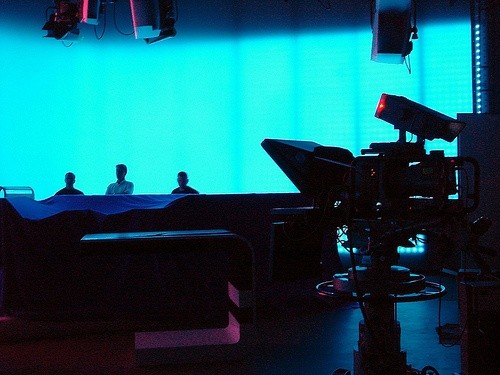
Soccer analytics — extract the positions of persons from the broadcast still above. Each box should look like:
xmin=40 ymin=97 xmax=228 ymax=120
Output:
xmin=105 ymin=164 xmax=134 ymax=194
xmin=56 ymin=172 xmax=83 ymax=195
xmin=171 ymin=171 xmax=198 ymax=194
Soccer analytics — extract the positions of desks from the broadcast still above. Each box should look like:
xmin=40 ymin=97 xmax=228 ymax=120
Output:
xmin=81 ymin=228 xmax=258 ymax=325
